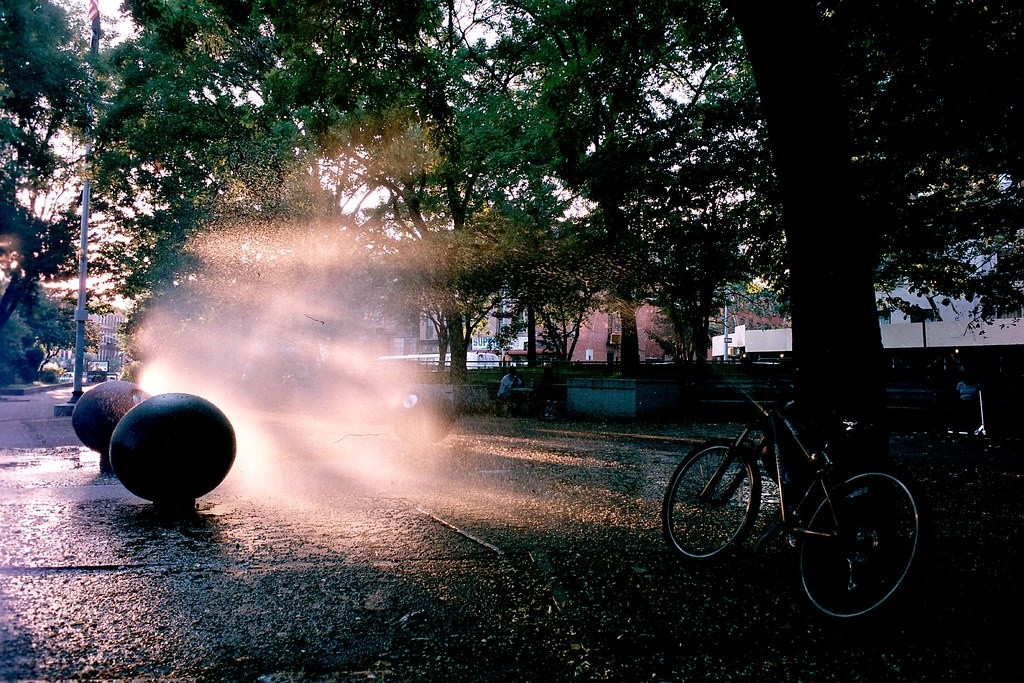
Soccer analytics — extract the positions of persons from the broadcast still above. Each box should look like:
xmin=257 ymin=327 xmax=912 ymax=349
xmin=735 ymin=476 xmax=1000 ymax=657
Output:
xmin=496 ymin=366 xmax=526 ymax=419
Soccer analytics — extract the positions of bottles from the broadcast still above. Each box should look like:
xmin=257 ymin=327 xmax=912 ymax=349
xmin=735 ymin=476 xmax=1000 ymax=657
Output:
xmin=530 ymin=379 xmax=533 ymax=388
xmin=525 ymin=383 xmax=528 ymax=388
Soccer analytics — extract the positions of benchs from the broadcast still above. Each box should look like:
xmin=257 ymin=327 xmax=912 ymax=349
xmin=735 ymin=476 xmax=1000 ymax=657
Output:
xmin=487 ymin=381 xmax=508 ymax=419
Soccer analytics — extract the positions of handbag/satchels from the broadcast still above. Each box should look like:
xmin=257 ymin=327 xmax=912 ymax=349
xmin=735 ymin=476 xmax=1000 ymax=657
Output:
xmin=543 ymin=399 xmax=557 ymax=420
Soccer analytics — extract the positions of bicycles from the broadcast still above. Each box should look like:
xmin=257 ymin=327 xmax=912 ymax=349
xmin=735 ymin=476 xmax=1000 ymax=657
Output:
xmin=662 ymin=381 xmax=927 ymax=629
